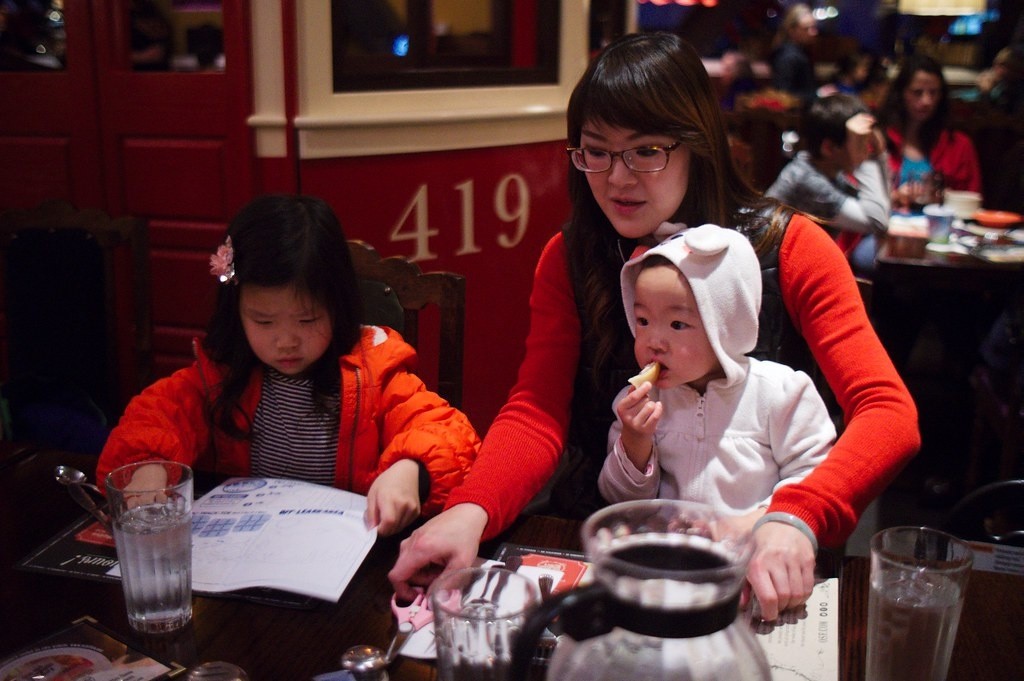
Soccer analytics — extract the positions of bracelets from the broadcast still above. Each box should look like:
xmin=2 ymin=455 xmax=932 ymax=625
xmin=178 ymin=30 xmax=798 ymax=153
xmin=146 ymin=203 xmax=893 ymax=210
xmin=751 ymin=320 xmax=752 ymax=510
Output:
xmin=752 ymin=512 xmax=818 ymax=558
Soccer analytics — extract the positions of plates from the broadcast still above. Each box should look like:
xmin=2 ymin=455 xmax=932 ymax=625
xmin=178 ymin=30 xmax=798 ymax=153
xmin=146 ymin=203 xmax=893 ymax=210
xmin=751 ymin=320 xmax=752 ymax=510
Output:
xmin=971 ymin=210 xmax=1023 ymax=228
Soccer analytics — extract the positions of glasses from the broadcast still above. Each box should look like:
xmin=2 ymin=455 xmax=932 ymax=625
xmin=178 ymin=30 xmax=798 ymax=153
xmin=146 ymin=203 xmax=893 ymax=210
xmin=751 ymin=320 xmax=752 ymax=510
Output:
xmin=565 ymin=137 xmax=685 ymax=173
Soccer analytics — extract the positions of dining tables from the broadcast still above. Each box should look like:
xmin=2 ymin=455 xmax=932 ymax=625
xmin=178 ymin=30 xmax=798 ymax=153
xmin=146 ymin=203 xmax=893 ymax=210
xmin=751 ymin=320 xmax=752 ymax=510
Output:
xmin=866 ymin=205 xmax=1024 ymax=497
xmin=0 ymin=440 xmax=1024 ymax=681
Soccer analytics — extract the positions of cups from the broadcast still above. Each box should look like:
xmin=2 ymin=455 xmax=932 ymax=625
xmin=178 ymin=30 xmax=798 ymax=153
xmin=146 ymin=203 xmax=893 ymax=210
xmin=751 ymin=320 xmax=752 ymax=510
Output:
xmin=432 ymin=567 xmax=536 ymax=681
xmin=923 ymin=208 xmax=955 ymax=245
xmin=866 ymin=527 xmax=973 ymax=680
xmin=105 ymin=460 xmax=192 ymax=631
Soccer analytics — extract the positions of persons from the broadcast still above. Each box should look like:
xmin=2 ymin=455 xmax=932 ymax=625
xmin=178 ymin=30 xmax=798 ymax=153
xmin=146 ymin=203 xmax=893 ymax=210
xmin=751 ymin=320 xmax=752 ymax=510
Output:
xmin=720 ymin=4 xmax=1024 ymax=373
xmin=26 ymin=7 xmax=225 ymax=73
xmin=389 ymin=29 xmax=920 ymax=620
xmin=98 ymin=193 xmax=485 ymax=536
xmin=598 ymin=225 xmax=836 ymax=548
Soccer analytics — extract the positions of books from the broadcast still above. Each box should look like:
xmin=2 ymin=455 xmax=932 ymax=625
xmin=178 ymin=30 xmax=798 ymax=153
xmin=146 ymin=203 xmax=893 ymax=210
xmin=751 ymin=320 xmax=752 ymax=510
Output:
xmin=106 ymin=475 xmax=380 ymax=603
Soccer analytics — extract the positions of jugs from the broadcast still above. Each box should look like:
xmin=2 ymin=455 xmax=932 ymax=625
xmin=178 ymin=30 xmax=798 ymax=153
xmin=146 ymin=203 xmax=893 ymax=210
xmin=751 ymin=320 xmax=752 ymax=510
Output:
xmin=513 ymin=499 xmax=771 ymax=680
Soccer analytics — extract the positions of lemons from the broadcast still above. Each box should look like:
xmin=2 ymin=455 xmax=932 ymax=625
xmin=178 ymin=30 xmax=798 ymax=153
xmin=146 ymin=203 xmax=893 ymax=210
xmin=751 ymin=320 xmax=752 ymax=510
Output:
xmin=628 ymin=361 xmax=659 ymax=389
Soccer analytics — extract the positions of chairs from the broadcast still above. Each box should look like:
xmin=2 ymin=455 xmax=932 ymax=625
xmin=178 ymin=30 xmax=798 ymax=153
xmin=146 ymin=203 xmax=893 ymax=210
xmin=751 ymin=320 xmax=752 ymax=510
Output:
xmin=965 ymin=293 xmax=1024 ymax=498
xmin=343 ymin=235 xmax=471 ymax=404
xmin=0 ymin=197 xmax=151 ymax=447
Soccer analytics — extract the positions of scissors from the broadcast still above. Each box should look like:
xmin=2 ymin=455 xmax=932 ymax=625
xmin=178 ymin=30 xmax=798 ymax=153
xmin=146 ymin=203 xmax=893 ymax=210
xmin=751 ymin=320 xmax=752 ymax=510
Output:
xmin=384 ymin=583 xmax=461 ymax=665
